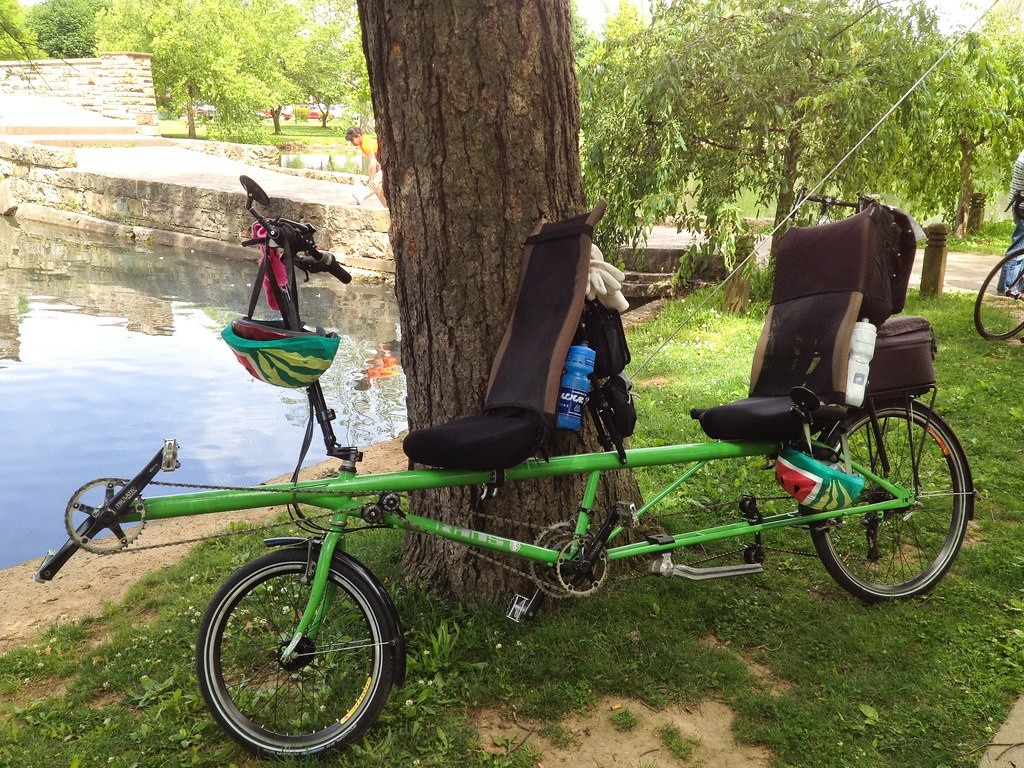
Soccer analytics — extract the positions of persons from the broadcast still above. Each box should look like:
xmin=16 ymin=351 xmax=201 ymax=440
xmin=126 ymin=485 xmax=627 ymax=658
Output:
xmin=345 ymin=127 xmax=388 ymax=208
xmin=997 ymin=150 xmax=1024 ymax=297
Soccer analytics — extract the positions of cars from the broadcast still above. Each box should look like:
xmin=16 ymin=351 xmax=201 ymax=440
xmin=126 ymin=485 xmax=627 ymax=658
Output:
xmin=193 ymin=103 xmax=215 ymax=120
xmin=329 ymin=105 xmax=359 ymax=120
xmin=259 ymin=106 xmax=292 ymax=120
xmin=295 ymin=104 xmax=334 ymax=122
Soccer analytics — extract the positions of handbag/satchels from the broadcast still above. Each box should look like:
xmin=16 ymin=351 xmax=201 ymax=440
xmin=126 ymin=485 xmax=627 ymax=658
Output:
xmin=852 ymin=319 xmax=938 ymax=410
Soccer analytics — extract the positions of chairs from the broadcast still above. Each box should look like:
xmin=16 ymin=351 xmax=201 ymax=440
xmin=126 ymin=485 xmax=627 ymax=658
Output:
xmin=403 ymin=197 xmax=608 ymax=471
xmin=701 ymin=199 xmax=897 ymax=441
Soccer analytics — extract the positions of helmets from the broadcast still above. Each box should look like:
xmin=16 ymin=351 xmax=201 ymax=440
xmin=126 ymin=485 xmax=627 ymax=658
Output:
xmin=221 ymin=317 xmax=341 ymax=388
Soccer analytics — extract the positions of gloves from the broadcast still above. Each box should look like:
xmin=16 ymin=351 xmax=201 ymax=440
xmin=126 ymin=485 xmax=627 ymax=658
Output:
xmin=585 ymin=243 xmax=630 ymax=316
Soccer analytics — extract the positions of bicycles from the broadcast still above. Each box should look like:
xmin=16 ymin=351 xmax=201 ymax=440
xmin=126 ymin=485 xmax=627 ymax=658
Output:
xmin=34 ymin=173 xmax=980 ymax=762
xmin=790 ymin=183 xmax=880 ymax=227
xmin=973 ymin=190 xmax=1024 ymax=341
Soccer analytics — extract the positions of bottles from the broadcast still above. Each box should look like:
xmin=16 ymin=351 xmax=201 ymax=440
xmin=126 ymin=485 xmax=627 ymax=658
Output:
xmin=556 ymin=341 xmax=596 ymax=432
xmin=845 ymin=318 xmax=877 ymax=406
